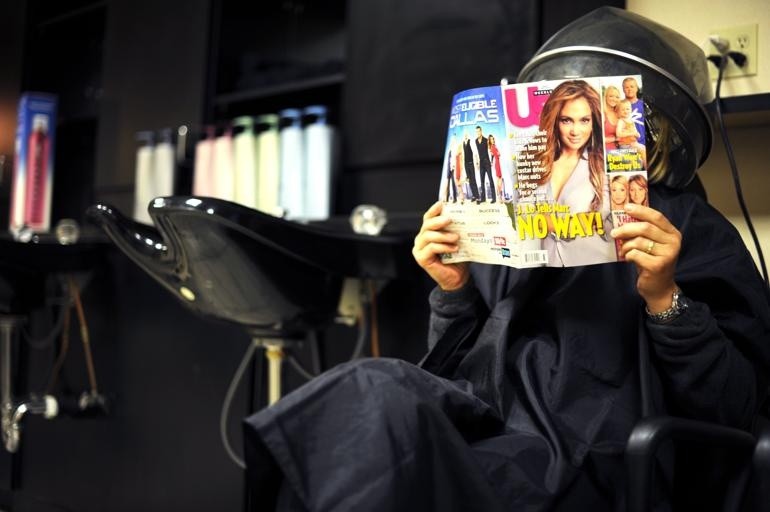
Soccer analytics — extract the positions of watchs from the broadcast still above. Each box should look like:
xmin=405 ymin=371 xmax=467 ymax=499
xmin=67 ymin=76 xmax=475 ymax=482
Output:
xmin=644 ymin=288 xmax=689 ymax=326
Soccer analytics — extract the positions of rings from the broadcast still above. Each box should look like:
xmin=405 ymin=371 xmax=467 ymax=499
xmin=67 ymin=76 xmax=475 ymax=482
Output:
xmin=647 ymin=241 xmax=656 ymax=255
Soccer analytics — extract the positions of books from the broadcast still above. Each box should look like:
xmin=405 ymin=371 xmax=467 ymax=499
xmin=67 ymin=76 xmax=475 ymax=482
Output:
xmin=431 ymin=74 xmax=648 ymax=272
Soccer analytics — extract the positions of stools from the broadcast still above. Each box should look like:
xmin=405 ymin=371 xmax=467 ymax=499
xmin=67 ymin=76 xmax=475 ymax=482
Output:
xmin=87 ymin=192 xmax=406 ymax=400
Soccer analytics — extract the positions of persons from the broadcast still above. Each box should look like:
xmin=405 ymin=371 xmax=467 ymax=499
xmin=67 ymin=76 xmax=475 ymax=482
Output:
xmin=620 ymin=78 xmax=645 ymax=150
xmin=625 ymin=175 xmax=649 ymax=210
xmin=609 ymin=175 xmax=629 ymax=212
xmin=512 ymin=82 xmax=603 ymax=214
xmin=614 ymin=103 xmax=645 ymax=173
xmin=600 ymin=85 xmax=623 ymax=158
xmin=241 ymin=14 xmax=768 ymax=505
xmin=441 ymin=127 xmax=508 ymax=206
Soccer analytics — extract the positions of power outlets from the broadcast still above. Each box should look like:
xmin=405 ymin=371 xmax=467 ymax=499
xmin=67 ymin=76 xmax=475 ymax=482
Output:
xmin=706 ymin=21 xmax=759 ymax=79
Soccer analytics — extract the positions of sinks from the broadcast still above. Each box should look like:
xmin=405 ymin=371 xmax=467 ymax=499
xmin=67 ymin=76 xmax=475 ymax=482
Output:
xmin=0 ymin=229 xmax=53 ymax=319
xmin=84 ymin=196 xmax=421 ymax=343
xmin=29 ymin=232 xmax=117 ymax=276
